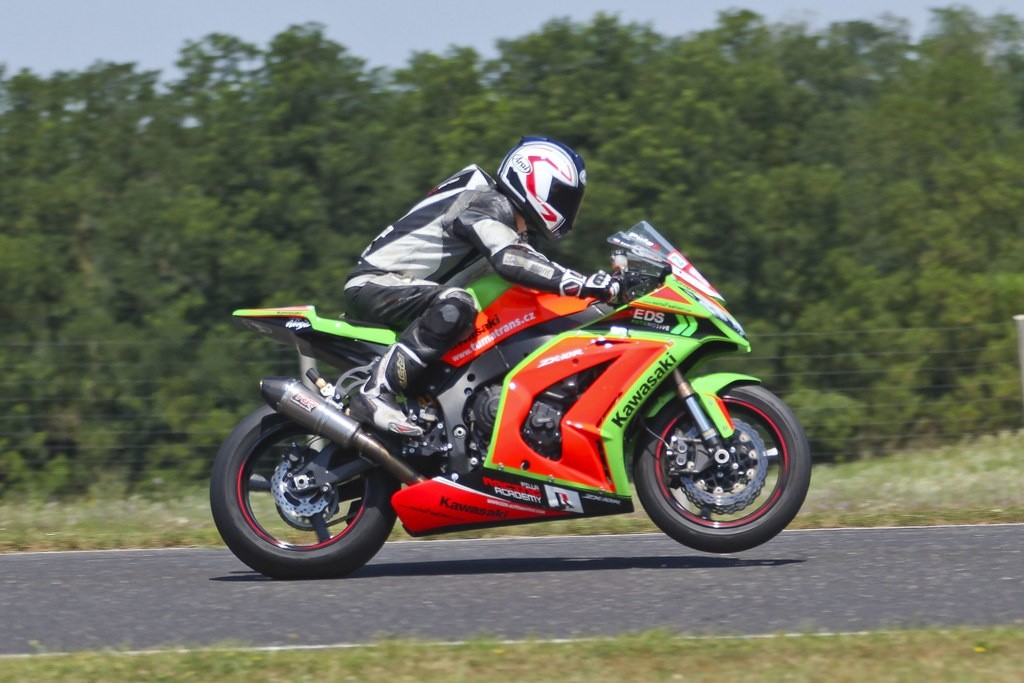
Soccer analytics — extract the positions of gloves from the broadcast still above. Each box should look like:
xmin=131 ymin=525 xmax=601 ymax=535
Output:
xmin=559 ymin=268 xmax=621 ymax=303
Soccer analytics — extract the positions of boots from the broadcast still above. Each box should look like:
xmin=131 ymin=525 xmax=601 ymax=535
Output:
xmin=349 ymin=341 xmax=425 ymax=436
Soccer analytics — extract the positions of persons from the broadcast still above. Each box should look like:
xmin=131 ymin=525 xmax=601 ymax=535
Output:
xmin=344 ymin=137 xmax=628 ymax=435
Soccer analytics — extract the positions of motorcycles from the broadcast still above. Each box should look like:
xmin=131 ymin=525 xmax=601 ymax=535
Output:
xmin=208 ymin=219 xmax=814 ymax=581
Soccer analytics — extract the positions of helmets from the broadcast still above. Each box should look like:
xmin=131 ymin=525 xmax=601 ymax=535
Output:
xmin=497 ymin=137 xmax=587 ymax=240
